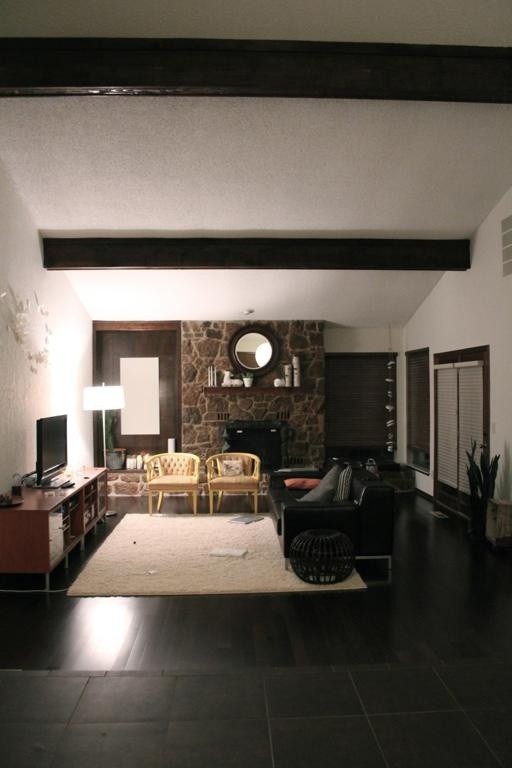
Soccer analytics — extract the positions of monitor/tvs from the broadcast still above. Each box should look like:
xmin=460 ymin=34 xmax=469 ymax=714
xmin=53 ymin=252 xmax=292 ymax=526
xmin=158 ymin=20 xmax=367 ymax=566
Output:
xmin=36 ymin=414 xmax=68 ymax=489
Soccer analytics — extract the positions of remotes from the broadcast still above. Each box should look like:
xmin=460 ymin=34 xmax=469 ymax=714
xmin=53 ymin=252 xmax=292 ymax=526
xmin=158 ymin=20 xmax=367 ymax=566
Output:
xmin=62 ymin=482 xmax=76 ymax=488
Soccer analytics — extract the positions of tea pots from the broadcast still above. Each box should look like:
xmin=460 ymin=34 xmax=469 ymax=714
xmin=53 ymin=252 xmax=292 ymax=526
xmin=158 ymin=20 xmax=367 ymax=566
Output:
xmin=359 ymin=458 xmax=378 ymax=473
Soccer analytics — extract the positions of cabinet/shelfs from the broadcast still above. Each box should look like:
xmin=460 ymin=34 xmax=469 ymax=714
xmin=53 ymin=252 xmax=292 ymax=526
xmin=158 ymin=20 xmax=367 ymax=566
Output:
xmin=0 ymin=467 xmax=109 ymax=589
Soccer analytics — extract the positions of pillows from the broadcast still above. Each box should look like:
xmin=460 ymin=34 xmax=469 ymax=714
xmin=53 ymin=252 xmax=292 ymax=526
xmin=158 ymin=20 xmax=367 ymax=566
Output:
xmin=284 ymin=464 xmax=352 ymax=502
xmin=223 ymin=460 xmax=244 ymax=477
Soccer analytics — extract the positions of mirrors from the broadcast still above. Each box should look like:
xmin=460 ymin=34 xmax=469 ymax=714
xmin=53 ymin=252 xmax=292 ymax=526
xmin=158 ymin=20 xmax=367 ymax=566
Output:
xmin=227 ymin=325 xmax=281 ymax=378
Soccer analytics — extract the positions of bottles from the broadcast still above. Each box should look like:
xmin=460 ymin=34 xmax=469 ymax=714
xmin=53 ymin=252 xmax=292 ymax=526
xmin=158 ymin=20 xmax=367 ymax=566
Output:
xmin=126 ymin=455 xmax=142 ymax=470
xmin=284 ymin=353 xmax=301 ymax=387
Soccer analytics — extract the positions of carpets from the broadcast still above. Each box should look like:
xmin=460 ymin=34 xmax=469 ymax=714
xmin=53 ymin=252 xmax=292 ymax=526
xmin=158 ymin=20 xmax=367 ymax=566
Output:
xmin=65 ymin=513 xmax=368 ymax=598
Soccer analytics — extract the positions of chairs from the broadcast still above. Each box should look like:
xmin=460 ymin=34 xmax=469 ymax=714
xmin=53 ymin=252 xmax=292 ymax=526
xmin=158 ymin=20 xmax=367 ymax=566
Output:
xmin=204 ymin=452 xmax=260 ymax=512
xmin=144 ymin=452 xmax=199 ymax=513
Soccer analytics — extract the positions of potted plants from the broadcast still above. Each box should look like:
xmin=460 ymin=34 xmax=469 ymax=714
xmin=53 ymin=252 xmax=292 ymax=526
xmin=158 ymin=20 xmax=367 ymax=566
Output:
xmin=465 ymin=435 xmax=501 ymax=544
xmin=100 ymin=409 xmax=127 ymax=470
xmin=241 ymin=372 xmax=255 ymax=388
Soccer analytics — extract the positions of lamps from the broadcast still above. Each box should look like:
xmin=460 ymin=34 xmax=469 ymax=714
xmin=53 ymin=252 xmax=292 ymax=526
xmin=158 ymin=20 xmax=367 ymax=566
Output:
xmin=83 ymin=382 xmax=125 ymax=516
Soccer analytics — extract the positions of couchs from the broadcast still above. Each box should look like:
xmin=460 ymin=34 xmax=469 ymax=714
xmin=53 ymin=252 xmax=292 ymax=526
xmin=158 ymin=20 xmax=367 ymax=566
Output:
xmin=267 ymin=458 xmax=395 ymax=570
